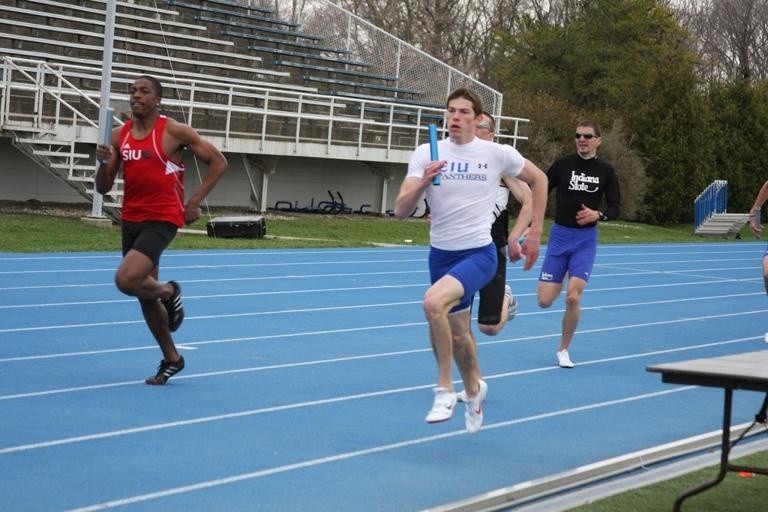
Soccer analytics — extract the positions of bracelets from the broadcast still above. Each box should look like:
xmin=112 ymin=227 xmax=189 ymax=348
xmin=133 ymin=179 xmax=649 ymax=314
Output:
xmin=596 ymin=211 xmax=605 ymax=221
xmin=750 ymin=215 xmax=757 ymax=218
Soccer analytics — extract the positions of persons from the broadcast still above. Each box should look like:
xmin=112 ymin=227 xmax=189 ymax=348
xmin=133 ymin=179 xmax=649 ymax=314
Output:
xmin=393 ymin=86 xmax=550 ymax=434
xmin=537 ymin=119 xmax=622 ymax=369
xmin=749 ymin=181 xmax=768 ymax=344
xmin=95 ymin=77 xmax=229 ymax=386
xmin=432 ymin=111 xmax=533 ymax=403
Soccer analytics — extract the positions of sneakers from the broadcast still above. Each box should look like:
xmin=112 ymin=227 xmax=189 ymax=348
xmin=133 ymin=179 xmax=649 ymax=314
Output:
xmin=425 ymin=385 xmax=458 ymax=424
xmin=556 ymin=349 xmax=575 ymax=369
xmin=160 ymin=280 xmax=185 ymax=333
xmin=457 ymin=377 xmax=488 ymax=436
xmin=504 ymin=283 xmax=518 ymax=322
xmin=145 ymin=352 xmax=185 ymax=386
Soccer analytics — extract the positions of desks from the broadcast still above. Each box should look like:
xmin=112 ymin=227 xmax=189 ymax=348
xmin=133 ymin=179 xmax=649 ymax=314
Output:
xmin=646 ymin=349 xmax=768 ymax=512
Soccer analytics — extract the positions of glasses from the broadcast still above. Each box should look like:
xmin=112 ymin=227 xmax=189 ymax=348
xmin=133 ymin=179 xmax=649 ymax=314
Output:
xmin=574 ymin=130 xmax=598 ymax=141
